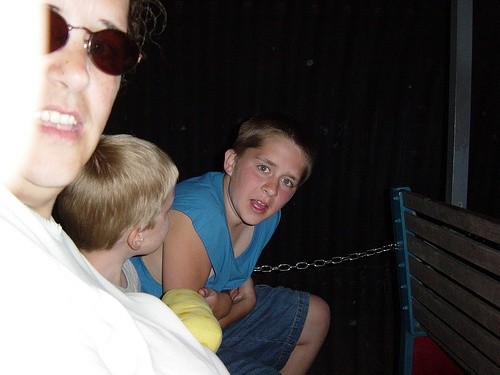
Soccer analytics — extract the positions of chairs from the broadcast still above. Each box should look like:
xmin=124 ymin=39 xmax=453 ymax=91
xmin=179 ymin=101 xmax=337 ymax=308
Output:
xmin=392 ymin=187 xmax=500 ymax=375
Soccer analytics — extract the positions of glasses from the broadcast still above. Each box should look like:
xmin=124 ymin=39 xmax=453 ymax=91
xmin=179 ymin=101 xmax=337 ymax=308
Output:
xmin=47 ymin=9 xmax=142 ymax=76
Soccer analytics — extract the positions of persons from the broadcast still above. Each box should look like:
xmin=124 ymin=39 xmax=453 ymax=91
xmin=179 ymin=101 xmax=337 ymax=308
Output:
xmin=130 ymin=115 xmax=330 ymax=375
xmin=51 ymin=134 xmax=179 ymax=292
xmin=0 ymin=0 xmax=230 ymax=375
